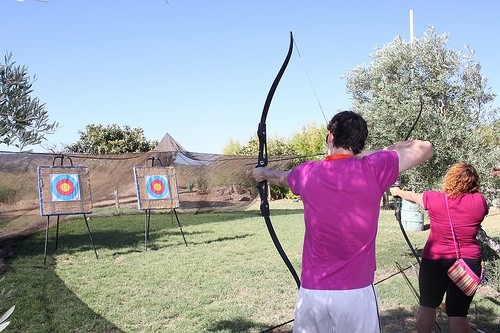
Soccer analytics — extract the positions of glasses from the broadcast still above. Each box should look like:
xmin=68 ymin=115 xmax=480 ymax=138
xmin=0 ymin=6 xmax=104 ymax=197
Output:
xmin=326 ymin=134 xmax=329 ymax=143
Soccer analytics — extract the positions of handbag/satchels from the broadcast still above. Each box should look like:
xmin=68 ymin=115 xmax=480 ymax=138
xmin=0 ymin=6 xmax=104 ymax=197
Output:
xmin=447 ymin=258 xmax=482 ymax=296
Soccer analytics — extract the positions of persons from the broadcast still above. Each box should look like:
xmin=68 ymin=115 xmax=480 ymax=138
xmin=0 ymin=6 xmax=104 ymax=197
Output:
xmin=251 ymin=110 xmax=435 ymax=333
xmin=389 ymin=162 xmax=490 ymax=333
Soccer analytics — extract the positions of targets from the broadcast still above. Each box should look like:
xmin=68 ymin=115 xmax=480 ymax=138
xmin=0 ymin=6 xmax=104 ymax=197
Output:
xmin=130 ymin=165 xmax=180 ymax=210
xmin=37 ymin=165 xmax=94 ymax=215
